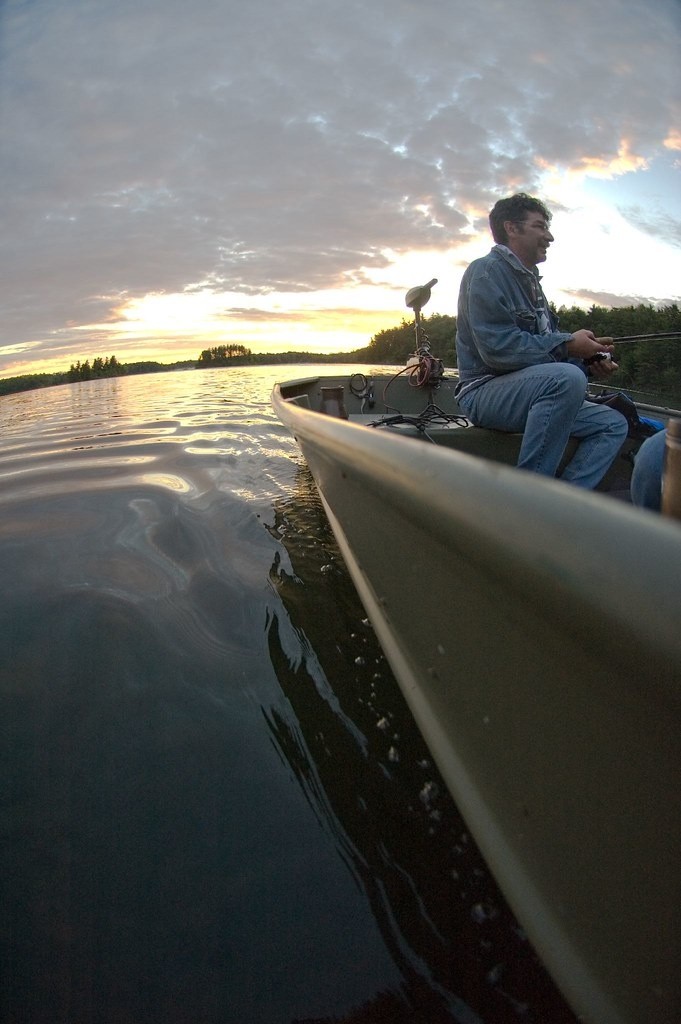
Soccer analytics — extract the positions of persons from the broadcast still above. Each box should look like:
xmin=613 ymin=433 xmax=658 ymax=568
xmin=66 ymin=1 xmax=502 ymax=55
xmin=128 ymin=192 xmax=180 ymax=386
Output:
xmin=454 ymin=193 xmax=627 ymax=492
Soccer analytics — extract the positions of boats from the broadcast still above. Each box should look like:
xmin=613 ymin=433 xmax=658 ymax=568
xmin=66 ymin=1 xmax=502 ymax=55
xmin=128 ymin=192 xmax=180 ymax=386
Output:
xmin=271 ymin=278 xmax=679 ymax=1024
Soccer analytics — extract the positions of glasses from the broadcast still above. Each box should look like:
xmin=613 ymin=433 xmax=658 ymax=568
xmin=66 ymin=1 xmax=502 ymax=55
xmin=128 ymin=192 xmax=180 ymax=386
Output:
xmin=512 ymin=220 xmax=550 ymax=231
